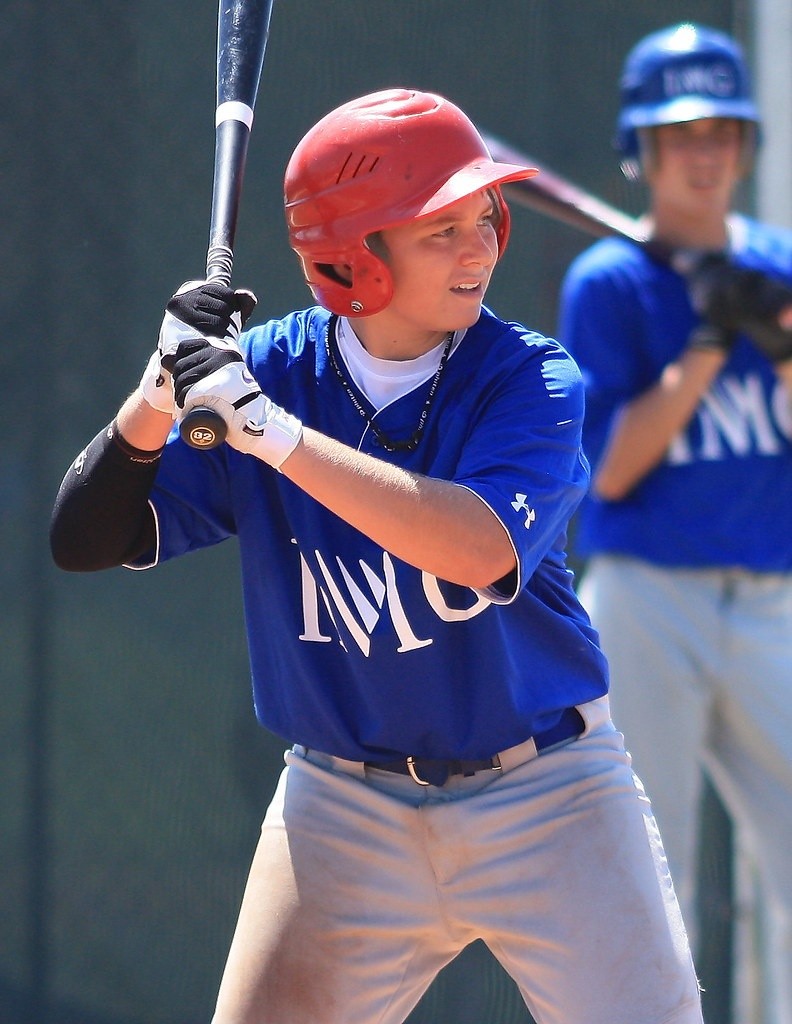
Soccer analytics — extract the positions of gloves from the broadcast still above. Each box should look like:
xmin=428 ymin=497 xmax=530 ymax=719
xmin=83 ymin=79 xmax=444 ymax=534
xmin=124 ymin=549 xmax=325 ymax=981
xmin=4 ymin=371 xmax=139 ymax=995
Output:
xmin=158 ymin=336 xmax=304 ymax=469
xmin=138 ymin=281 xmax=258 ymax=415
xmin=690 ymin=254 xmax=790 ymax=359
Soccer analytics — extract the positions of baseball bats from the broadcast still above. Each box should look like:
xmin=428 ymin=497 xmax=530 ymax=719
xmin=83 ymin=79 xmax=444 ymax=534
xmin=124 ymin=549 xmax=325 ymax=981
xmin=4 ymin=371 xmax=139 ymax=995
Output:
xmin=482 ymin=133 xmax=792 ymax=330
xmin=179 ymin=1 xmax=273 ymax=450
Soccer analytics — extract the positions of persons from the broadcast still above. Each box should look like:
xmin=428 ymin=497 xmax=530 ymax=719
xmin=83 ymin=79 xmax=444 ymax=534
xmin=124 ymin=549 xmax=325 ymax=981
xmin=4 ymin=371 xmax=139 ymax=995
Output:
xmin=48 ymin=89 xmax=702 ymax=1024
xmin=558 ymin=21 xmax=792 ymax=1023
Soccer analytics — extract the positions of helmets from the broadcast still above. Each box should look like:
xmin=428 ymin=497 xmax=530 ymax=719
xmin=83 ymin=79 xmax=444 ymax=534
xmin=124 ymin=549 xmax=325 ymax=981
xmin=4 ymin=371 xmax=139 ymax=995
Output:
xmin=283 ymin=88 xmax=541 ymax=318
xmin=614 ymin=20 xmax=763 ymax=181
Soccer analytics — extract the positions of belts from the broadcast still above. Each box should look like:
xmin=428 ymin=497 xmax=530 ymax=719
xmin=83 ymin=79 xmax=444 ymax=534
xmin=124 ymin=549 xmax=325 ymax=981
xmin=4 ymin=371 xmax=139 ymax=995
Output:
xmin=366 ymin=710 xmax=585 ymax=785
